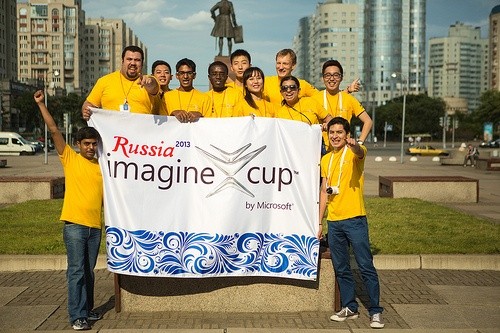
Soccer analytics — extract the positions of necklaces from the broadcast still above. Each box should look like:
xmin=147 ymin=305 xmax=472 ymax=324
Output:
xmin=212 ymin=89 xmax=226 ymax=118
xmin=325 ymin=93 xmax=340 ymax=117
xmin=254 ymin=100 xmax=267 ymax=117
xmin=285 ymin=101 xmax=303 ymax=121
xmin=178 ymin=89 xmax=194 ymax=112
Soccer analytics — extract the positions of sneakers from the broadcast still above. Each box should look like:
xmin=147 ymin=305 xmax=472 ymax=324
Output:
xmin=87 ymin=310 xmax=102 ymax=320
xmin=72 ymin=318 xmax=91 ymax=330
xmin=329 ymin=307 xmax=358 ymax=321
xmin=370 ymin=313 xmax=385 ymax=328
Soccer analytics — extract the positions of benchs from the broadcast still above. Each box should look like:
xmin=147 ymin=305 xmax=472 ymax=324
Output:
xmin=476 ymin=158 xmax=500 ymax=170
xmin=378 ymin=176 xmax=480 ymax=203
xmin=0 ymin=175 xmax=65 ymax=204
xmin=439 ymin=158 xmax=477 ymax=165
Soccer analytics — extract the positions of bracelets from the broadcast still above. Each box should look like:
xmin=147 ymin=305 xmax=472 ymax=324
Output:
xmin=347 ymin=87 xmax=352 ymax=93
xmin=319 ymin=224 xmax=322 ymax=225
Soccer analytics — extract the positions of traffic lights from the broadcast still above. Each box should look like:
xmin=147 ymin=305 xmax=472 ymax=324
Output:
xmin=447 ymin=117 xmax=451 ymax=126
xmin=438 ymin=117 xmax=443 ymax=128
xmin=63 ymin=113 xmax=66 ymax=127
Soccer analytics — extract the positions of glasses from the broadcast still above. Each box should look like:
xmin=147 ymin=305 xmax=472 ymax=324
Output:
xmin=176 ymin=71 xmax=194 ymax=78
xmin=323 ymin=72 xmax=342 ymax=79
xmin=282 ymin=85 xmax=298 ymax=92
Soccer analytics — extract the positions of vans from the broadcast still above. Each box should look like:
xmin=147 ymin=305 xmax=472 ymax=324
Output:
xmin=0 ymin=132 xmax=36 ymax=156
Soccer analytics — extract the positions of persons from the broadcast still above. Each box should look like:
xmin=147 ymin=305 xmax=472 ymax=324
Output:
xmin=205 ymin=61 xmax=247 ymax=118
xmin=231 ymin=49 xmax=252 ymax=87
xmin=159 ymin=58 xmax=212 ymax=122
xmin=274 ymin=76 xmax=333 ymax=131
xmin=210 ymin=0 xmax=237 ymax=56
xmin=237 ymin=66 xmax=276 ymax=119
xmin=463 ymin=144 xmax=479 ymax=168
xmin=81 ymin=46 xmax=162 ymax=118
xmin=304 ymin=59 xmax=374 ymax=145
xmin=34 ymin=90 xmax=104 ymax=330
xmin=262 ymin=48 xmax=361 ymax=107
xmin=316 ymin=117 xmax=385 ymax=329
xmin=151 ymin=60 xmax=174 ymax=97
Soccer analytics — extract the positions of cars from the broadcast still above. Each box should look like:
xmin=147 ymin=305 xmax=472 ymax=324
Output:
xmin=405 ymin=144 xmax=449 ymax=156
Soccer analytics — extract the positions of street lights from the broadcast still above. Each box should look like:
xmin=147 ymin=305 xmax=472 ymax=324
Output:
xmin=44 ymin=70 xmax=60 ymax=165
xmin=391 ymin=72 xmax=409 ymax=164
